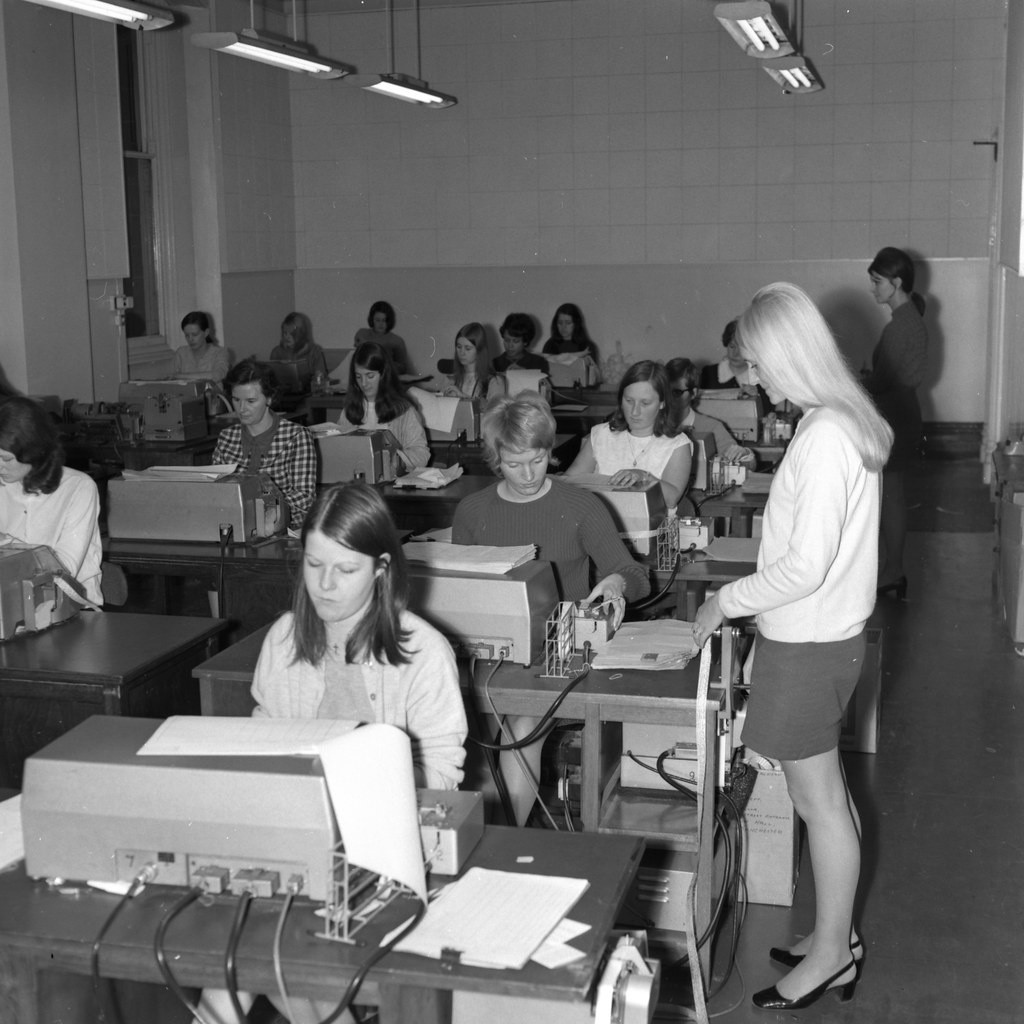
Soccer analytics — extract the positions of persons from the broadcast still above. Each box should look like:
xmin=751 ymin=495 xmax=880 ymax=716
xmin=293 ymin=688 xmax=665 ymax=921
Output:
xmin=269 ymin=311 xmax=326 ymax=393
xmin=492 ymin=313 xmax=554 ymax=407
xmin=0 ymin=396 xmax=104 ymax=612
xmin=191 ymin=483 xmax=469 ymax=1024
xmin=859 ymin=247 xmax=928 ymax=599
xmin=563 ymin=359 xmax=693 ymax=517
xmin=698 ymin=281 xmax=895 ymax=1011
xmin=664 ymin=357 xmax=757 ymax=472
xmin=336 ymin=342 xmax=431 ymax=477
xmin=203 ymin=357 xmax=316 ymax=620
xmin=355 ymin=301 xmax=407 ymax=376
xmin=700 ymin=321 xmax=776 ymax=417
xmin=452 ymin=400 xmax=650 ymax=828
xmin=168 ymin=311 xmax=230 ymax=417
xmin=430 ymin=322 xmax=505 ymax=414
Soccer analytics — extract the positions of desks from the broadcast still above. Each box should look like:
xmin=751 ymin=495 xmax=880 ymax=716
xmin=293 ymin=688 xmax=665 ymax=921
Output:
xmin=0 ymin=388 xmax=805 ymax=1024
xmin=991 ymin=450 xmax=1024 ymax=643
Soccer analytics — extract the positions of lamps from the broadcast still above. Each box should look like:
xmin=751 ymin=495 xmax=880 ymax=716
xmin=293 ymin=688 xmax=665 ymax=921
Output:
xmin=360 ymin=0 xmax=459 ymax=110
xmin=713 ymin=0 xmax=796 ymax=58
xmin=22 ymin=0 xmax=174 ymax=32
xmin=760 ymin=0 xmax=823 ymax=94
xmin=189 ymin=0 xmax=358 ymax=80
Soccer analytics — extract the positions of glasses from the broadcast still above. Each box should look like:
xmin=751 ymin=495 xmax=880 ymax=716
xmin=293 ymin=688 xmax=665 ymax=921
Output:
xmin=672 ymin=388 xmax=690 ymax=398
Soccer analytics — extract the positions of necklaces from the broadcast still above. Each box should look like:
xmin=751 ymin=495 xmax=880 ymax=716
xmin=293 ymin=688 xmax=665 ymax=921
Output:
xmin=543 ymin=303 xmax=603 ymax=389
xmin=627 ymin=433 xmax=652 ymax=467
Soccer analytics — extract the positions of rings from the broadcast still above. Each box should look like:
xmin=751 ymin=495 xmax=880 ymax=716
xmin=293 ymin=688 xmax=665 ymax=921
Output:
xmin=693 ymin=629 xmax=697 ymax=635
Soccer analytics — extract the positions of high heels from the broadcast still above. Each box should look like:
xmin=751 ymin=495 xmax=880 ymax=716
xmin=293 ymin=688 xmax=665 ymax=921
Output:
xmin=877 ymin=576 xmax=908 ymax=599
xmin=769 ymin=939 xmax=864 ymax=983
xmin=753 ymin=959 xmax=858 ymax=1010
